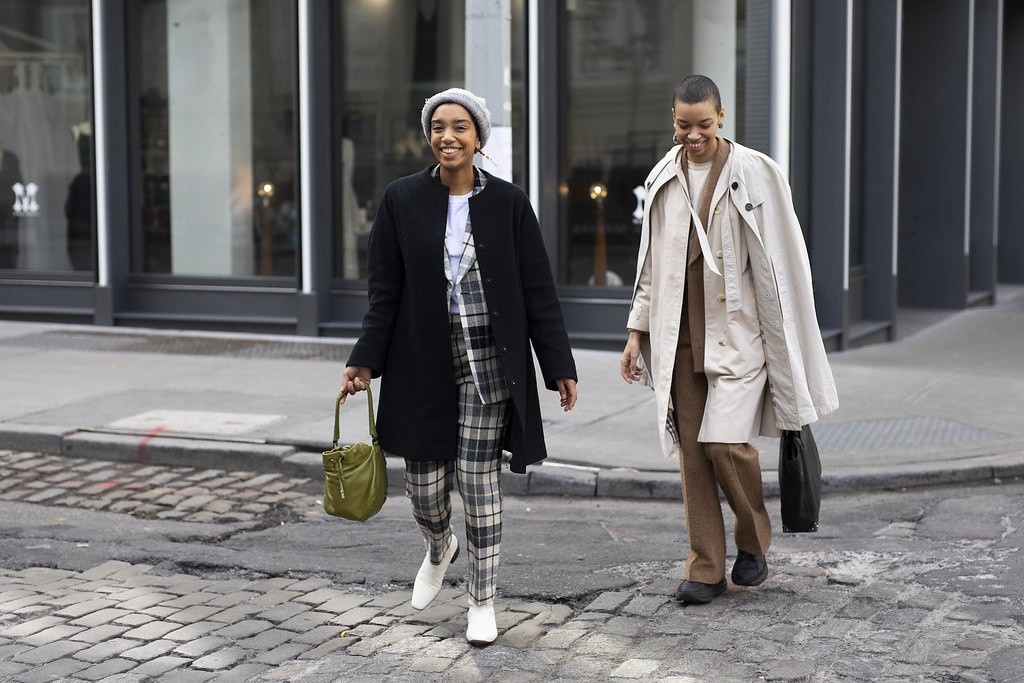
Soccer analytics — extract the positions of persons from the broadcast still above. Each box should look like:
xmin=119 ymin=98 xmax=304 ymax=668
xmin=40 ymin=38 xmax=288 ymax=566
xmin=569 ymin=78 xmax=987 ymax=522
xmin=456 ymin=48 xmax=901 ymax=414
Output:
xmin=620 ymin=76 xmax=838 ymax=602
xmin=340 ymin=89 xmax=579 ymax=651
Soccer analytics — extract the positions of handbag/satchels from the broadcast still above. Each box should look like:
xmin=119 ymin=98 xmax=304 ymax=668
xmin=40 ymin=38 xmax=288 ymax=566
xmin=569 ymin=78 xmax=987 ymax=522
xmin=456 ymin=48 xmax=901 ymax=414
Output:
xmin=778 ymin=424 xmax=822 ymax=534
xmin=322 ymin=379 xmax=389 ymax=521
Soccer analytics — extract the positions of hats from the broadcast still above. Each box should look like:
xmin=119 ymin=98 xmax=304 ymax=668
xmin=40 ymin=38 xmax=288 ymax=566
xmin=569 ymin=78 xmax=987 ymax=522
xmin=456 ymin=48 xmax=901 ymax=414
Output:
xmin=420 ymin=87 xmax=492 ymax=155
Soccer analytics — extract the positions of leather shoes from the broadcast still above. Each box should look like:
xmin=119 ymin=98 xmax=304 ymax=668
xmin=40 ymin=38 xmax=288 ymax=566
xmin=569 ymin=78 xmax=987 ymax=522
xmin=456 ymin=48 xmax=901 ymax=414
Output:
xmin=410 ymin=534 xmax=460 ymax=611
xmin=467 ymin=601 xmax=499 ymax=648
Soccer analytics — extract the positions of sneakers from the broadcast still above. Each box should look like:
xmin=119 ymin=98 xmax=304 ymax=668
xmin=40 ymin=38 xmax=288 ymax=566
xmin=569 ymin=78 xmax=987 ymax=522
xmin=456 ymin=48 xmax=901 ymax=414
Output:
xmin=730 ymin=549 xmax=770 ymax=587
xmin=673 ymin=577 xmax=727 ymax=604
